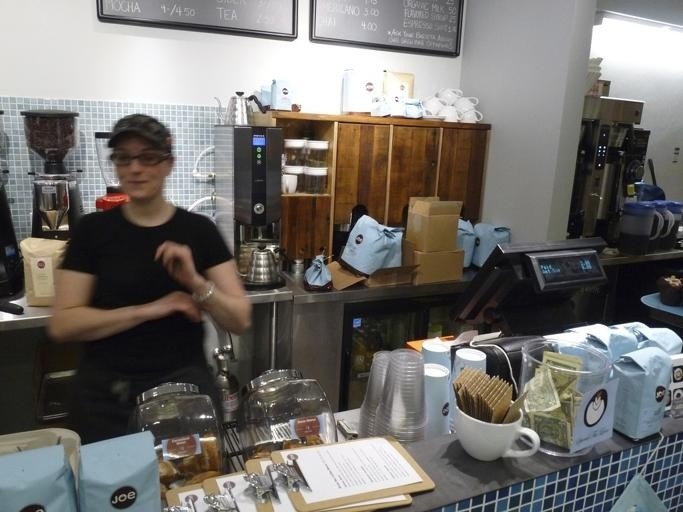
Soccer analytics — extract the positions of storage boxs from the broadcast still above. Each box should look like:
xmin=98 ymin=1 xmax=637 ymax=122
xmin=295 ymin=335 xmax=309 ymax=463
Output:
xmin=406 ymin=197 xmax=463 ymax=252
xmin=413 ymin=248 xmax=465 ymax=285
xmin=327 ymin=239 xmax=421 ymax=291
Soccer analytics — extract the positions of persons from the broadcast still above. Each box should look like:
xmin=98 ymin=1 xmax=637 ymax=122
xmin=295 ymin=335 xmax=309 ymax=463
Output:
xmin=47 ymin=113 xmax=254 ymax=444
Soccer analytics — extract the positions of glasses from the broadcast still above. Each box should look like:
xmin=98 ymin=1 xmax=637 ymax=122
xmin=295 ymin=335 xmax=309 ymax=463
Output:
xmin=108 ymin=150 xmax=173 ymax=166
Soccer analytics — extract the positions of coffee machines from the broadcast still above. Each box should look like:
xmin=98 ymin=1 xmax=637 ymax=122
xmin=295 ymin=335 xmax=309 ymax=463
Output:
xmin=215 ymin=125 xmax=286 ymax=290
xmin=20 ymin=109 xmax=85 ymax=241
xmin=93 ymin=131 xmax=130 ymax=214
xmin=0 ymin=110 xmax=25 ymax=304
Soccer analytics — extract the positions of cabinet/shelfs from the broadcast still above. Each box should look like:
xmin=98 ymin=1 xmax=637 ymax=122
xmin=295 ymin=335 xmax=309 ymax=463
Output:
xmin=275 ymin=117 xmax=338 ymax=263
xmin=388 ymin=127 xmax=444 ymax=228
xmin=438 ymin=128 xmax=487 ymax=221
xmin=335 ymin=122 xmax=391 ymax=224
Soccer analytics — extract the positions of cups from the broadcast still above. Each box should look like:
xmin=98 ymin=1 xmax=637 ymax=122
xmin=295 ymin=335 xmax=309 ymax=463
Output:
xmin=359 ymin=339 xmax=541 ymax=462
xmin=422 ymin=88 xmax=483 ymax=124
xmin=617 ymin=200 xmax=682 ymax=255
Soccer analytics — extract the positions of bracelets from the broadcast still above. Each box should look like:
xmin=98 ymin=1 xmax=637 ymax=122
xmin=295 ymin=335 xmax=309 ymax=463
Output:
xmin=191 ymin=280 xmax=215 ymax=303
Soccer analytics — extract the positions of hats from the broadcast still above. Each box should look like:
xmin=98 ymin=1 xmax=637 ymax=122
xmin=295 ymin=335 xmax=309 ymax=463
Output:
xmin=107 ymin=114 xmax=171 ymax=152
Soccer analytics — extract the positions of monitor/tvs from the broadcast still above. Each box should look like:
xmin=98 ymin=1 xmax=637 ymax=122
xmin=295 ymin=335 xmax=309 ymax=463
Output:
xmin=447 ymin=235 xmax=608 ymax=337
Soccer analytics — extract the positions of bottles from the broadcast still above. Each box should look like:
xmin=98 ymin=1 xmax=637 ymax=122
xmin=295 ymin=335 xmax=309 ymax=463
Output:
xmin=520 ymin=339 xmax=612 ymax=459
xmin=235 ymin=369 xmax=338 ymax=462
xmin=129 ymin=382 xmax=224 ymax=506
xmin=283 ymin=139 xmax=330 ymax=195
xmin=270 ymin=79 xmax=277 ymax=108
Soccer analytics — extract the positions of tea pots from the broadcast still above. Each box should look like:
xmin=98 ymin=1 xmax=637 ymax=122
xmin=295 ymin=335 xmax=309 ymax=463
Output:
xmin=214 ymin=91 xmax=266 ymax=126
